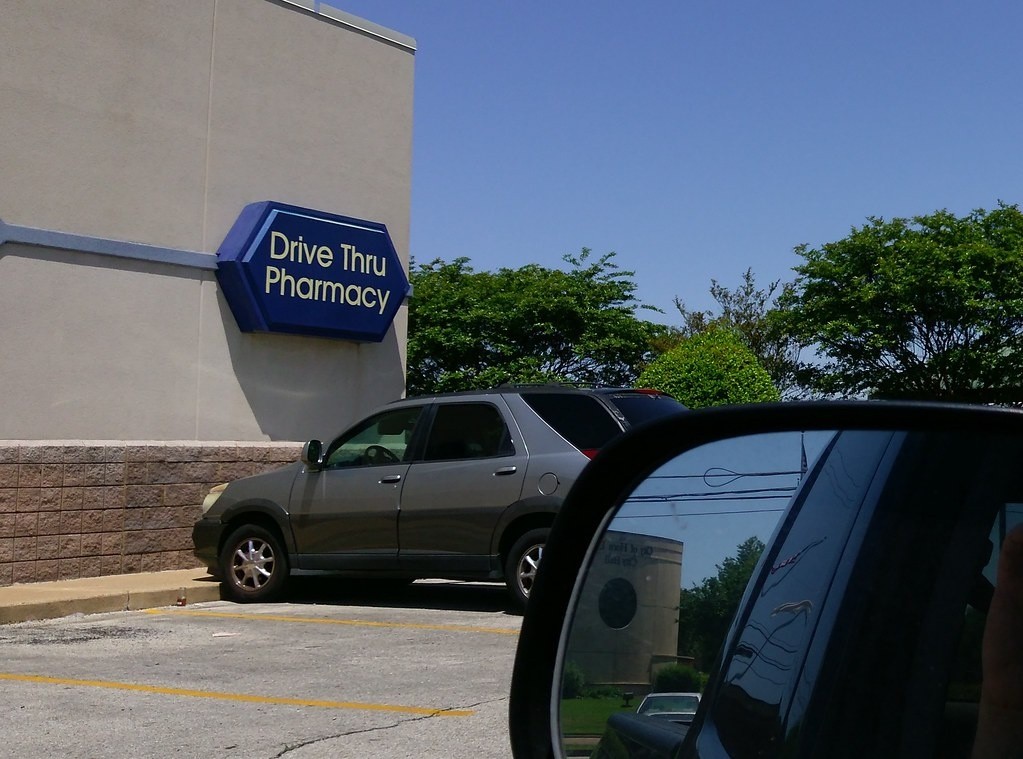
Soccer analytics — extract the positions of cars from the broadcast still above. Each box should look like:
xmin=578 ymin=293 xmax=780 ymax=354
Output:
xmin=637 ymin=693 xmax=703 ymax=728
xmin=192 ymin=386 xmax=698 ymax=619
